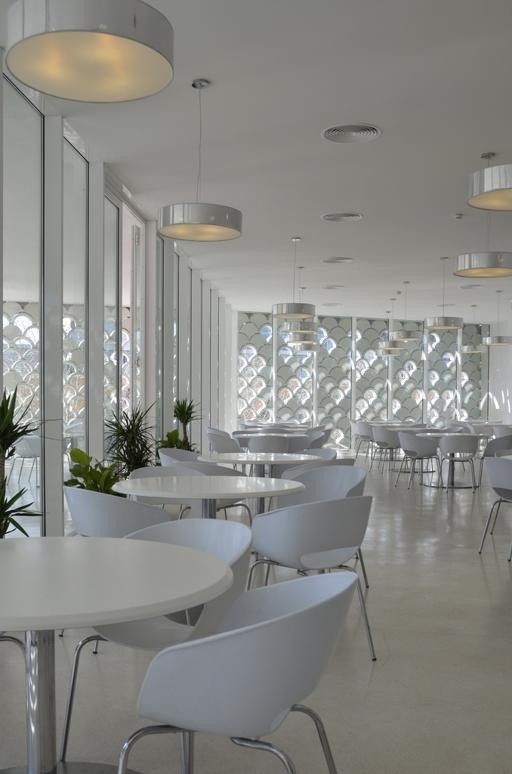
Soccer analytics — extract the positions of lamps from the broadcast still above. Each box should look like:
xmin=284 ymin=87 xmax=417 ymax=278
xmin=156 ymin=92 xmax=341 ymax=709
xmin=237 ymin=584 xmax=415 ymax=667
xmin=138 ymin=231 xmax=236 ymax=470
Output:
xmin=155 ymin=79 xmax=244 ymax=241
xmin=451 ymin=153 xmax=512 ymax=278
xmin=377 ymin=309 xmax=407 ymax=351
xmin=388 ymin=282 xmax=425 ymax=346
xmin=284 ymin=284 xmax=319 ymax=346
xmin=483 ymin=288 xmax=511 ymax=349
xmin=0 ymin=0 xmax=177 ymax=106
xmin=461 ymin=164 xmax=512 ymax=206
xmin=271 ymin=238 xmax=318 ymax=321
xmin=425 ymin=255 xmax=461 ymax=331
xmin=463 ymin=304 xmax=487 ymax=355
xmin=280 ymin=267 xmax=319 ymax=334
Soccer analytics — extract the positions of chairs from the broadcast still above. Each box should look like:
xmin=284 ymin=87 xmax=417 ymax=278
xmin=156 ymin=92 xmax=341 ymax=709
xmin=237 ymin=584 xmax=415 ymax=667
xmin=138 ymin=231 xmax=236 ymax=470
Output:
xmin=246 ymin=496 xmax=382 ymax=665
xmin=478 ymin=453 xmax=512 ymax=559
xmin=60 ymin=515 xmax=253 ymax=771
xmin=275 ymin=463 xmax=378 ymax=589
xmin=208 ymin=416 xmax=330 ymax=445
xmin=117 ymin=567 xmax=362 ymax=774
xmin=368 ymin=424 xmax=394 ymax=475
xmin=126 ymin=466 xmax=206 ymax=515
xmin=270 ymin=448 xmax=336 ymax=474
xmin=401 ymin=421 xmax=512 ymax=432
xmin=61 ymin=484 xmax=178 ymax=537
xmin=157 ymin=446 xmax=216 ymax=466
xmin=437 ymin=433 xmax=481 ymax=493
xmin=394 ymin=433 xmax=432 ymax=489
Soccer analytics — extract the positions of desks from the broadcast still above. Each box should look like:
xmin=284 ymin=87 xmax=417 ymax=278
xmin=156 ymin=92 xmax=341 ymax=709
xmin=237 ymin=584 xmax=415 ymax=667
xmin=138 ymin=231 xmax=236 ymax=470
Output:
xmin=195 ymin=446 xmax=325 ymax=470
xmin=113 ymin=472 xmax=294 ymax=509
xmin=0 ymin=531 xmax=236 ymax=774
